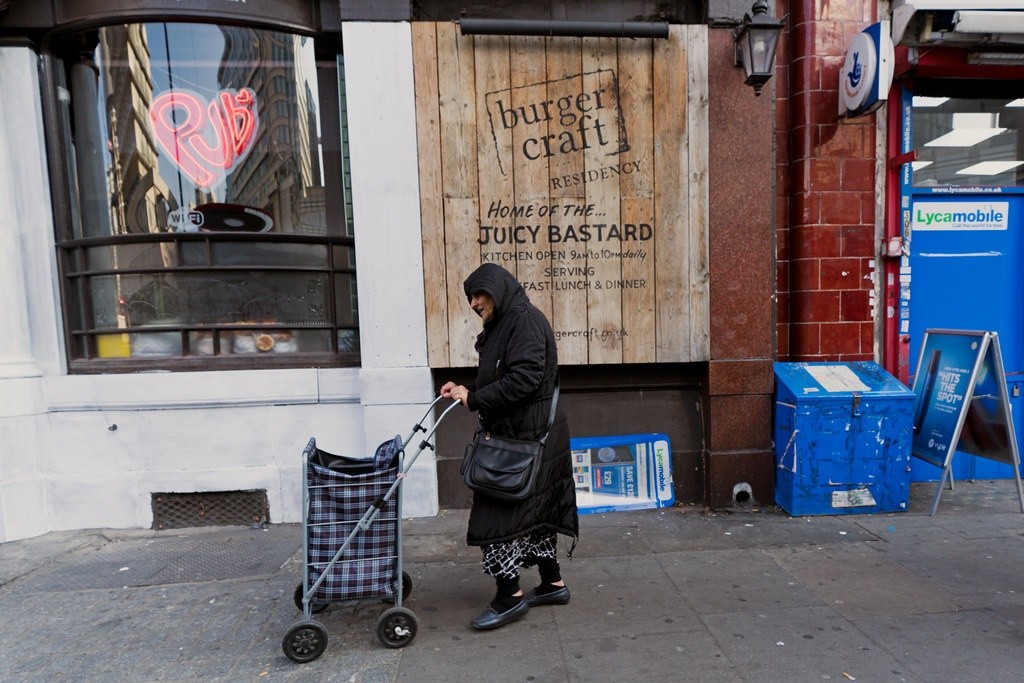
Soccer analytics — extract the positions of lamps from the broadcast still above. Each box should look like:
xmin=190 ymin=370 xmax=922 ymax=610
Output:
xmin=732 ymin=0 xmax=785 ymax=96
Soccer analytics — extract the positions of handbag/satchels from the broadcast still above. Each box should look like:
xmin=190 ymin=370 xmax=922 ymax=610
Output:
xmin=461 ymin=427 xmax=546 ymax=504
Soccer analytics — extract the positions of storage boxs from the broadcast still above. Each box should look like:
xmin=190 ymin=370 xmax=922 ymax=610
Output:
xmin=774 ymin=361 xmax=917 ymax=516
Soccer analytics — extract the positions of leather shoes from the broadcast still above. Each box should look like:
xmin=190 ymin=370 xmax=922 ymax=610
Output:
xmin=522 ymin=587 xmax=570 ymax=607
xmin=470 ymin=598 xmax=528 ymax=629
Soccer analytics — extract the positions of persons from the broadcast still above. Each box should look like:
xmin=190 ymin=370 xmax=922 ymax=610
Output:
xmin=441 ymin=263 xmax=579 ymax=631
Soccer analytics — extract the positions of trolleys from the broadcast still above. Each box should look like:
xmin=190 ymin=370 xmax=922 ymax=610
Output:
xmin=279 ymin=394 xmax=461 ymax=664
